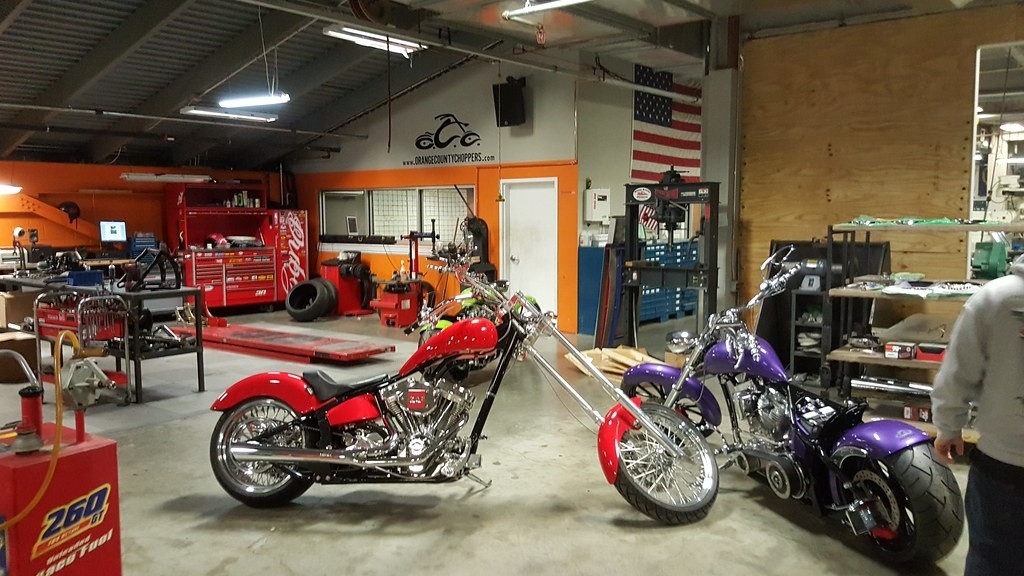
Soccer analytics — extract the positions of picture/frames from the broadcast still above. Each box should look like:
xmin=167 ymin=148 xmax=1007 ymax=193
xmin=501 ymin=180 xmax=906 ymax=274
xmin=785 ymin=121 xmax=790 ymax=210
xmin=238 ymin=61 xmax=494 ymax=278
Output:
xmin=347 ymin=215 xmax=359 ymax=235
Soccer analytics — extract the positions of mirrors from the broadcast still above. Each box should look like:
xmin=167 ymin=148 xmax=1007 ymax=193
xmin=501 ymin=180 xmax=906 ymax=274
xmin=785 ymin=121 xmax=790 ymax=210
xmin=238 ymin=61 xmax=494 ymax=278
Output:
xmin=965 ymin=40 xmax=1024 ymax=281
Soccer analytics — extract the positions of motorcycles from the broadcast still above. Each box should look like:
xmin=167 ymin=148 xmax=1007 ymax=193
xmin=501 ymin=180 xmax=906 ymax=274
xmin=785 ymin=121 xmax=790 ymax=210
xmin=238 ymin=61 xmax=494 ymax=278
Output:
xmin=619 ymin=243 xmax=965 ymax=571
xmin=208 ymin=216 xmax=721 ymax=525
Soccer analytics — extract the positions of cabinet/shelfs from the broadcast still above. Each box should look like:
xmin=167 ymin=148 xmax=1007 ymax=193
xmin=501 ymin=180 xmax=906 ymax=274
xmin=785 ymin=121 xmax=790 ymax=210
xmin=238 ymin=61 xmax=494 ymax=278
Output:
xmin=790 ymin=291 xmax=828 ymax=385
xmin=826 ymin=221 xmax=1020 ymax=436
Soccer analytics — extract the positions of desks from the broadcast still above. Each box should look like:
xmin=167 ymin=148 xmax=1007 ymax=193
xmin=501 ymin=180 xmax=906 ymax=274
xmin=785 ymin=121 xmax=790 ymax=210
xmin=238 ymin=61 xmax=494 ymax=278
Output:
xmin=80 ymin=255 xmax=138 ymax=278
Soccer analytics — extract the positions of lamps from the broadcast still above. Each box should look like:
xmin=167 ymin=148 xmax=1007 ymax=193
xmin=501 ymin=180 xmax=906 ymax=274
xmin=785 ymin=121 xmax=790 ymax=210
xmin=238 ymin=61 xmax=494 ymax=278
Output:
xmin=178 ymin=104 xmax=280 ymax=124
xmin=322 ymin=22 xmax=431 ymax=55
xmin=217 ymin=85 xmax=289 ymax=113
xmin=120 ymin=170 xmax=214 ymax=184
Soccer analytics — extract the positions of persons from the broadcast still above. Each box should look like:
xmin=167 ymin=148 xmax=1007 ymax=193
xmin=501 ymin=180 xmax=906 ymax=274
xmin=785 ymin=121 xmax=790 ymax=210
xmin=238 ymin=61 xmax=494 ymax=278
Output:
xmin=930 ymin=252 xmax=1024 ymax=576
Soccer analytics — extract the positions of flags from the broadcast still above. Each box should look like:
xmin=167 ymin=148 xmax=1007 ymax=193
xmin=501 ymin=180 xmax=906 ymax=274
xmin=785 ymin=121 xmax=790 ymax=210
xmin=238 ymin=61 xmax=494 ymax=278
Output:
xmin=630 ymin=62 xmax=703 ymax=185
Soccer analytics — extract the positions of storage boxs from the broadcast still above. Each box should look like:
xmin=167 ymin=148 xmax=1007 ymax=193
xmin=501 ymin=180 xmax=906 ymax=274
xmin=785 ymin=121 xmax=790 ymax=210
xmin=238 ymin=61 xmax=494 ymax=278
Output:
xmin=0 ymin=286 xmax=47 ymax=332
xmin=0 ymin=332 xmax=42 ymax=384
xmin=885 ymin=333 xmax=961 ymax=425
